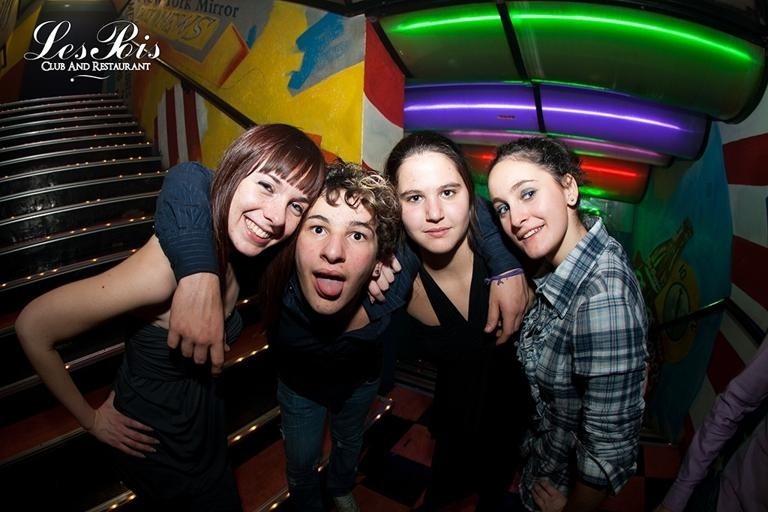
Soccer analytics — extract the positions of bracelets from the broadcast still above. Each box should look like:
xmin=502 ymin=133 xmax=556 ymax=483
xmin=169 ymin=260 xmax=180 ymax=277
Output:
xmin=483 ymin=270 xmax=525 ymax=284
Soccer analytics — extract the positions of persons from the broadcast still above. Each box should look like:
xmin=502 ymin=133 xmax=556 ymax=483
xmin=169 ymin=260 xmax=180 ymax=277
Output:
xmin=15 ymin=125 xmax=402 ymax=512
xmin=388 ymin=131 xmax=537 ymax=511
xmin=154 ymin=156 xmax=539 ymax=511
xmin=654 ymin=343 xmax=766 ymax=512
xmin=486 ymin=137 xmax=651 ymax=512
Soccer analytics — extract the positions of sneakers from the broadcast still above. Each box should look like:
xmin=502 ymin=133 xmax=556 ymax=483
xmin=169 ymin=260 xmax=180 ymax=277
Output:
xmin=335 ymin=493 xmax=358 ymax=512
